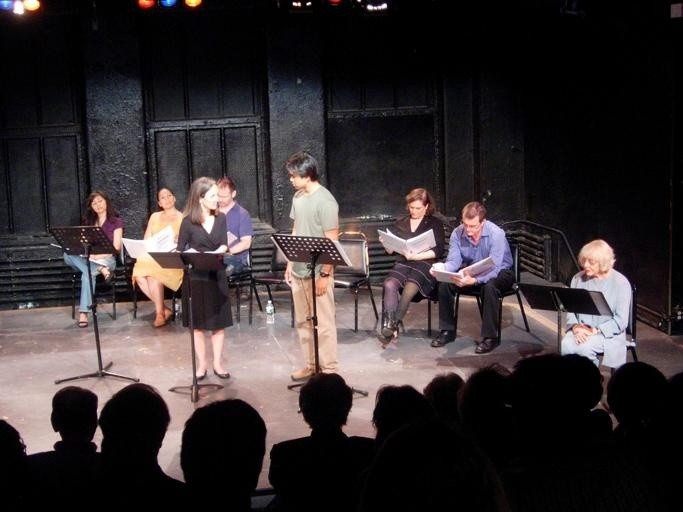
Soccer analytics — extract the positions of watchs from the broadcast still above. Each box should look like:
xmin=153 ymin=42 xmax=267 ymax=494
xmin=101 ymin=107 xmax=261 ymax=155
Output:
xmin=319 ymin=271 xmax=330 ymax=278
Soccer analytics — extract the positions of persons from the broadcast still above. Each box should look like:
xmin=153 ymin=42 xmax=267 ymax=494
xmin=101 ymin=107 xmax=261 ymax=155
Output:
xmin=377 ymin=187 xmax=446 ymax=349
xmin=430 ymin=201 xmax=516 ymax=354
xmin=63 ymin=191 xmax=124 ymax=328
xmin=131 ymin=186 xmax=190 ymax=328
xmin=284 ymin=151 xmax=339 ymax=381
xmin=215 ymin=175 xmax=254 ymax=277
xmin=174 ymin=176 xmax=234 ymax=381
xmin=0 ymin=352 xmax=682 ymax=511
xmin=561 ymin=238 xmax=632 ymax=414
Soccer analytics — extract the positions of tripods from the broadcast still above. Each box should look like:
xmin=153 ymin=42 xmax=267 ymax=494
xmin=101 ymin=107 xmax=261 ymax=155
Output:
xmin=53 ymin=259 xmax=141 ymax=387
xmin=169 ymin=275 xmax=224 ymax=403
xmin=287 ymin=263 xmax=369 ymax=414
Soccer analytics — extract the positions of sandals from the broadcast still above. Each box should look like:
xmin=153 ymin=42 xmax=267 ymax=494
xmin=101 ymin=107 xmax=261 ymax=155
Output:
xmin=77 ymin=311 xmax=88 ymax=328
xmin=99 ymin=267 xmax=117 ymax=288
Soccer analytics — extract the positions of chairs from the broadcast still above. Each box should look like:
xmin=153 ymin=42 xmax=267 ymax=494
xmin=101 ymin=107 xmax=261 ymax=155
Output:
xmin=452 ymin=244 xmax=529 ymax=346
xmin=332 ymin=230 xmax=382 ymax=330
xmin=72 ymin=234 xmax=129 ymax=322
xmin=596 ymin=284 xmax=638 ymax=372
xmin=248 ymin=229 xmax=298 ymax=325
xmin=225 ymin=234 xmax=261 ymax=322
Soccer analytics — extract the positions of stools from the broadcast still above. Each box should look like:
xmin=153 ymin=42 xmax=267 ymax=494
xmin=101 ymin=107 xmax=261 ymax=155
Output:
xmin=390 ymin=288 xmax=438 ymax=335
xmin=132 ymin=279 xmax=185 ymax=321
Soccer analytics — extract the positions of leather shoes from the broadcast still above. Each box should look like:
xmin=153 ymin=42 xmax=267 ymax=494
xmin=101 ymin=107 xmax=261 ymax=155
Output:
xmin=474 ymin=337 xmax=499 ymax=353
xmin=212 ymin=368 xmax=229 ymax=379
xmin=152 ymin=308 xmax=173 ymax=327
xmin=195 ymin=368 xmax=207 ymax=380
xmin=431 ymin=329 xmax=455 ymax=347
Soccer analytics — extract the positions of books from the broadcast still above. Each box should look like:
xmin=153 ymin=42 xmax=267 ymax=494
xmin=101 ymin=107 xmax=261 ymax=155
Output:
xmin=377 ymin=227 xmax=437 ymax=260
xmin=119 ymin=225 xmax=178 ymax=261
xmin=434 ymin=257 xmax=496 ymax=284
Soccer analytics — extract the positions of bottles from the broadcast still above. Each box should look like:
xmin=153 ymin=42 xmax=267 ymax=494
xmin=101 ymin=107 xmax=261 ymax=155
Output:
xmin=173 ymin=298 xmax=180 ymax=320
xmin=265 ymin=300 xmax=275 ymax=324
xmin=13 ymin=302 xmax=40 ymax=310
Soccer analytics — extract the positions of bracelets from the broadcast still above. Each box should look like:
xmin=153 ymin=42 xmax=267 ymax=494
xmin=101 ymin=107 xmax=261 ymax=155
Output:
xmin=572 ymin=324 xmax=578 ymax=330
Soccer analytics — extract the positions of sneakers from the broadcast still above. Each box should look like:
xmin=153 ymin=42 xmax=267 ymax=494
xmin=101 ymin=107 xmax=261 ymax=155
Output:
xmin=290 ymin=366 xmax=313 ymax=380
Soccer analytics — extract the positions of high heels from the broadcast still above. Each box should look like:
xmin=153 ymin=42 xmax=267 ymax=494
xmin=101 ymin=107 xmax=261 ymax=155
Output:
xmin=376 ymin=309 xmax=400 ymax=349
